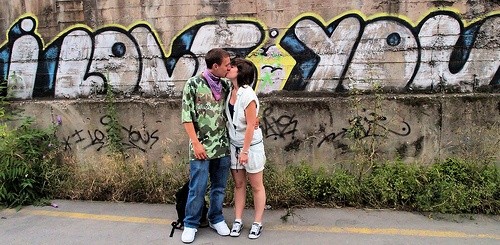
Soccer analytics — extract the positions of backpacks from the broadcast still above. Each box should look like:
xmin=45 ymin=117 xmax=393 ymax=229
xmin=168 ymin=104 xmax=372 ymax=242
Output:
xmin=175 ymin=180 xmax=209 ymax=228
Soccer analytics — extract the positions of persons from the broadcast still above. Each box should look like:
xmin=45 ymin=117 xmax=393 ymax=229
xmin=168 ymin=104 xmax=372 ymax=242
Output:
xmin=181 ymin=47 xmax=259 ymax=244
xmin=225 ymin=57 xmax=267 ymax=239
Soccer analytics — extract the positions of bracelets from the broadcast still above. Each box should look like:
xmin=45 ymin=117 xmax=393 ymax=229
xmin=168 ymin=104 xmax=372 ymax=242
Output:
xmin=241 ymin=150 xmax=250 ymax=155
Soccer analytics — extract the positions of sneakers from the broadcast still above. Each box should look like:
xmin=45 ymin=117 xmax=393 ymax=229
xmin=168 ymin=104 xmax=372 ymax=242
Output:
xmin=181 ymin=227 xmax=197 ymax=243
xmin=209 ymin=220 xmax=230 ymax=235
xmin=230 ymin=221 xmax=244 ymax=236
xmin=249 ymin=223 xmax=263 ymax=239
